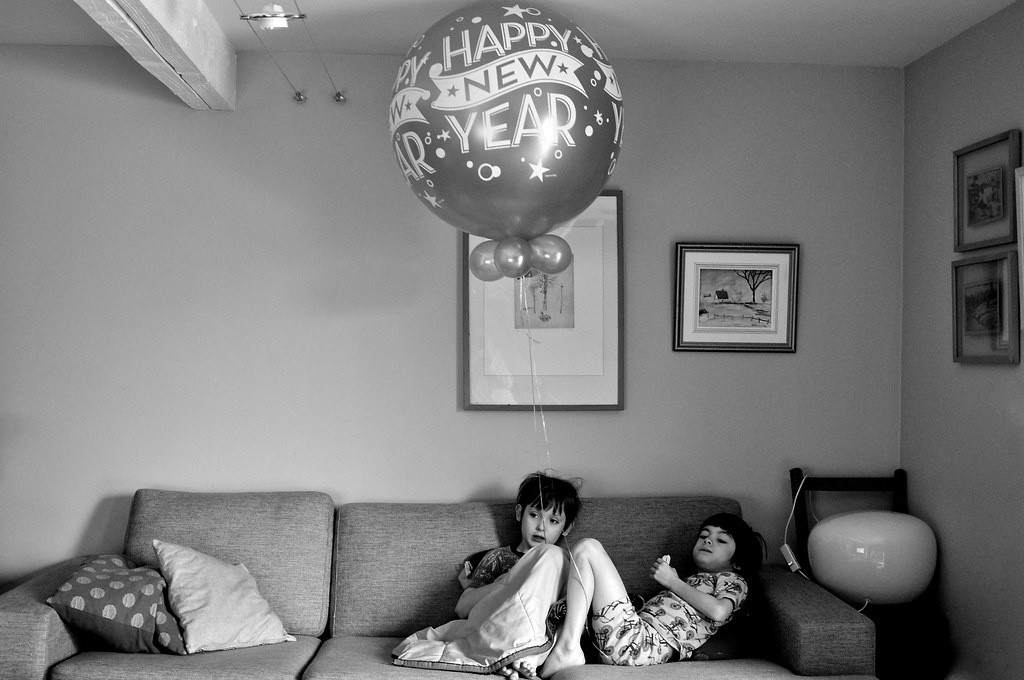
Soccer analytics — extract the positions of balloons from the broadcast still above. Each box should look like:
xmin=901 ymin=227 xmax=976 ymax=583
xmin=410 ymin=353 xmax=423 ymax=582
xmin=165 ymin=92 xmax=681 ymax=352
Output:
xmin=530 ymin=235 xmax=573 ymax=275
xmin=470 ymin=240 xmax=504 ymax=282
xmin=389 ymin=0 xmax=625 ymax=241
xmin=494 ymin=238 xmax=533 ymax=278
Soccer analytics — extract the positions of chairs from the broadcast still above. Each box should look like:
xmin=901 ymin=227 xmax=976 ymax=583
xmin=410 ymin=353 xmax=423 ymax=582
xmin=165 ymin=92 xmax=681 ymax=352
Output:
xmin=788 ymin=467 xmax=908 ymax=563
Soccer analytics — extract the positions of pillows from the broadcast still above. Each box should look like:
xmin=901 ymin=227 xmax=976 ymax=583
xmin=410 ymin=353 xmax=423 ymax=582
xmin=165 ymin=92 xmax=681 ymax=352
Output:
xmin=45 ymin=553 xmax=187 ymax=656
xmin=151 ymin=538 xmax=297 ymax=654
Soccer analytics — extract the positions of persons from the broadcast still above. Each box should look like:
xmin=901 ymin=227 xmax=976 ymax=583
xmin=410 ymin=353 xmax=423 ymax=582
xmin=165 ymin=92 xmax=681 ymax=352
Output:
xmin=539 ymin=512 xmax=761 ymax=678
xmin=455 ymin=474 xmax=580 ymax=680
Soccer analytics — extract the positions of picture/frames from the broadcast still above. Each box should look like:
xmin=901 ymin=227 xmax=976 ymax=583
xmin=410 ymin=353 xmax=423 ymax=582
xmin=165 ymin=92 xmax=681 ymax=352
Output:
xmin=672 ymin=241 xmax=801 ymax=354
xmin=951 ymin=250 xmax=1021 ymax=365
xmin=952 ymin=129 xmax=1022 ymax=253
xmin=461 ymin=189 xmax=624 ymax=412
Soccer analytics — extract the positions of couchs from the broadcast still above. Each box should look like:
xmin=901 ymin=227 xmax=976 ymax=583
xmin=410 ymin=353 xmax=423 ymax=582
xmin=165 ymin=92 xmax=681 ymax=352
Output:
xmin=0 ymin=488 xmax=881 ymax=680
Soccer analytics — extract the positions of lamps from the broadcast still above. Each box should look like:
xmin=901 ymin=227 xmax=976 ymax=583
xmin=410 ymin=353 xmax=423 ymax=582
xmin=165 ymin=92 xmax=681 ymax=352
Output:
xmin=807 ymin=509 xmax=937 ymax=605
